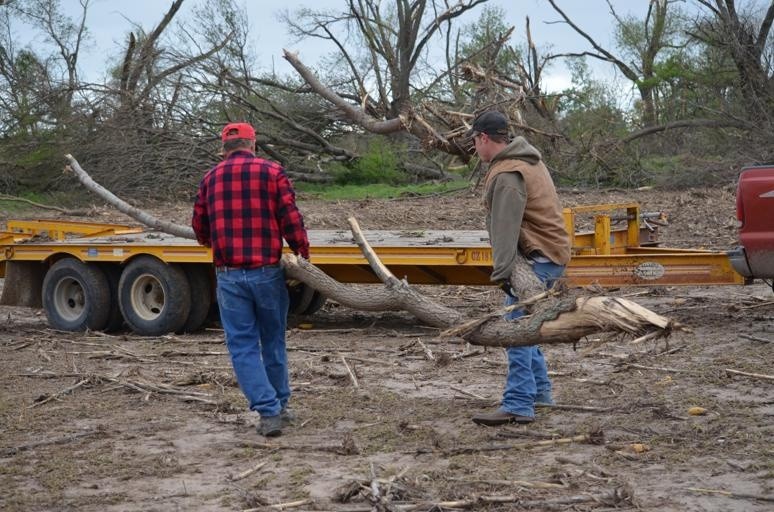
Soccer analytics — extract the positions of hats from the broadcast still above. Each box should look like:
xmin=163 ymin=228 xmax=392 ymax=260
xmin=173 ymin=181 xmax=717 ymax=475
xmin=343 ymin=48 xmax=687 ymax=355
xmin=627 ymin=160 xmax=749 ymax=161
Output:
xmin=463 ymin=113 xmax=508 ymax=139
xmin=222 ymin=123 xmax=256 ymax=142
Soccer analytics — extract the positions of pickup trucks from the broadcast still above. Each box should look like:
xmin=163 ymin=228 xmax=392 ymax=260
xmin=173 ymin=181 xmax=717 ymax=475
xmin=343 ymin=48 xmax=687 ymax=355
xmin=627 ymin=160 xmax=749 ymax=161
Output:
xmin=735 ymin=164 xmax=773 ymax=299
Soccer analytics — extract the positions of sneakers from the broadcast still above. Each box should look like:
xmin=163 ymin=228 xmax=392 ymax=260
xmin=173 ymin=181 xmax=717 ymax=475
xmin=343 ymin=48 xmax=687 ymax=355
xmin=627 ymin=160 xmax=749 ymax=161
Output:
xmin=255 ymin=409 xmax=294 ymax=436
xmin=472 ymin=409 xmax=535 ymax=424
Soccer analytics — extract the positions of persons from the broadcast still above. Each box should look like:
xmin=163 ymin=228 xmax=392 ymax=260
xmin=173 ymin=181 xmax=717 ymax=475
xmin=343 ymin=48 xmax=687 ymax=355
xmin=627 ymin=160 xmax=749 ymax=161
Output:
xmin=464 ymin=109 xmax=571 ymax=426
xmin=190 ymin=122 xmax=309 ymax=438
